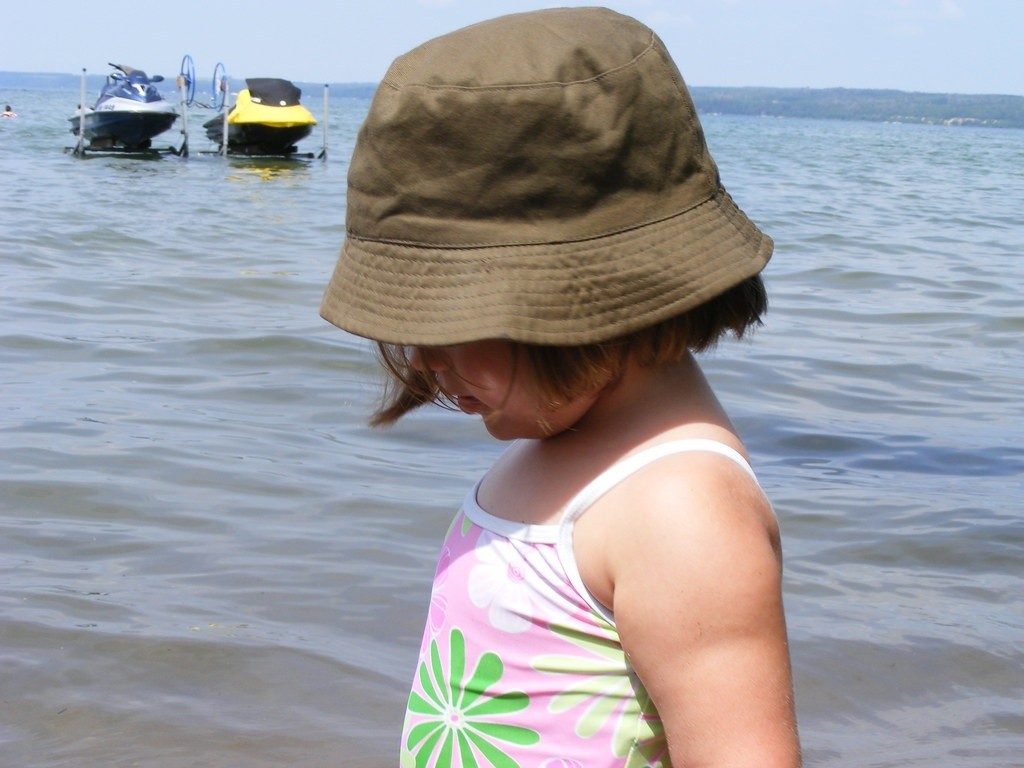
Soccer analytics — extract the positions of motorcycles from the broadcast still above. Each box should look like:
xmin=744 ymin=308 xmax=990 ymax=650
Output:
xmin=203 ymin=64 xmax=330 ymax=164
xmin=66 ymin=54 xmax=198 ymax=158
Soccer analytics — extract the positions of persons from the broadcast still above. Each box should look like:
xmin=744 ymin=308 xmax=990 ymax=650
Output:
xmin=318 ymin=7 xmax=809 ymax=768
xmin=1 ymin=106 xmax=16 ymax=118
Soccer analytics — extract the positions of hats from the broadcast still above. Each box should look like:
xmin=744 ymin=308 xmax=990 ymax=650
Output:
xmin=320 ymin=6 xmax=773 ymax=346
